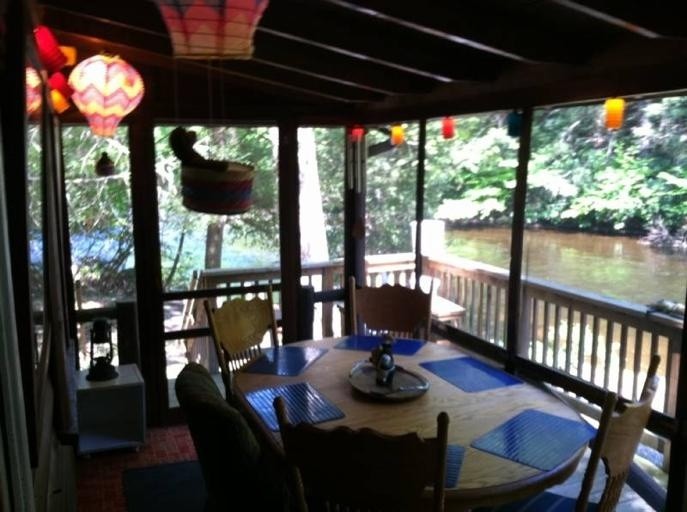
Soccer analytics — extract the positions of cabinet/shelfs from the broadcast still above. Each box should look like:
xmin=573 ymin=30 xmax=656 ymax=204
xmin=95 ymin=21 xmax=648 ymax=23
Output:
xmin=76 ymin=362 xmax=145 ymax=454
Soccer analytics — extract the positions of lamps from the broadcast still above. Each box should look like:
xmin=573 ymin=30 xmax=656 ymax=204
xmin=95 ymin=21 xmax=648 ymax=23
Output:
xmin=86 ymin=317 xmax=119 ymax=382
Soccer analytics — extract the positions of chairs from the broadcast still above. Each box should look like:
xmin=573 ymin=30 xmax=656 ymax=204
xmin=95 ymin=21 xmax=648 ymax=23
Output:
xmin=174 ymin=275 xmax=661 ymax=511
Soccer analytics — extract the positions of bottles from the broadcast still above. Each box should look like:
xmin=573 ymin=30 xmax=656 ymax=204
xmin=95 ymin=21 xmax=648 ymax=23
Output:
xmin=377 ymin=329 xmax=396 ymax=389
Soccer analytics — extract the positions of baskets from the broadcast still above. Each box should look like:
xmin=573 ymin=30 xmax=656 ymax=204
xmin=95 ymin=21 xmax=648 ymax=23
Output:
xmin=181 ymin=159 xmax=255 ymax=215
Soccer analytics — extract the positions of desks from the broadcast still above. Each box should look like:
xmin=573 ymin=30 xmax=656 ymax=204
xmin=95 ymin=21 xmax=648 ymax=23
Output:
xmin=230 ymin=331 xmax=590 ymax=508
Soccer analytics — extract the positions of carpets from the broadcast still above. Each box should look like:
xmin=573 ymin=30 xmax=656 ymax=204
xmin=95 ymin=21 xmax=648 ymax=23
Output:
xmin=75 ymin=423 xmax=199 ymax=511
